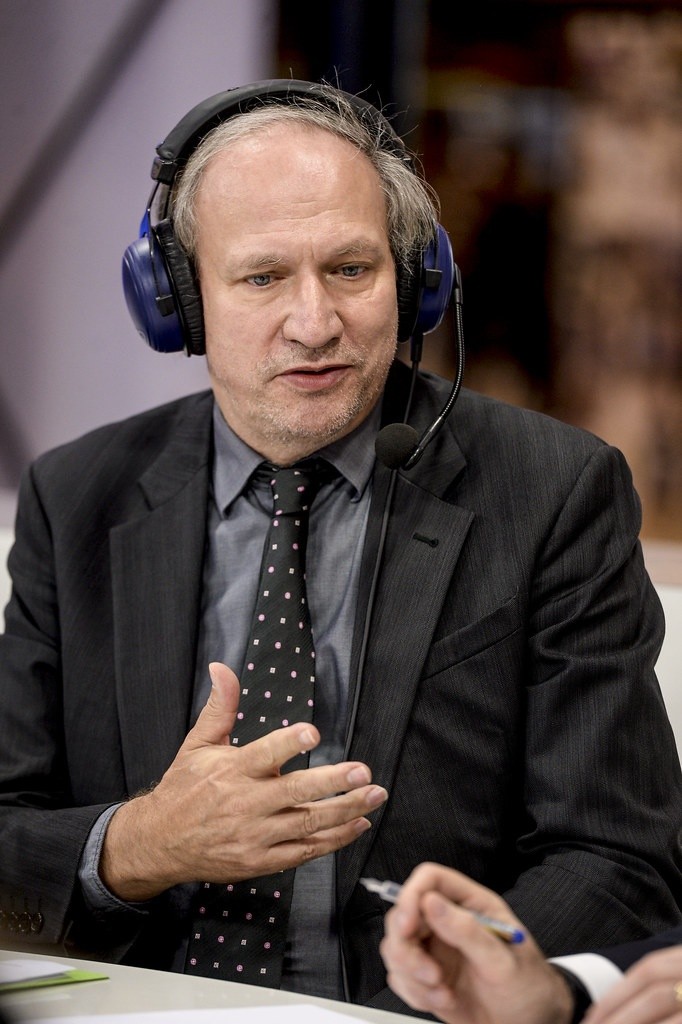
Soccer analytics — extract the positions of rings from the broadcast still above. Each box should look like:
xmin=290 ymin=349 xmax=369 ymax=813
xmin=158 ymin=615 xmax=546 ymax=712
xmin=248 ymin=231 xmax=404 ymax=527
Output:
xmin=674 ymin=983 xmax=682 ymax=1000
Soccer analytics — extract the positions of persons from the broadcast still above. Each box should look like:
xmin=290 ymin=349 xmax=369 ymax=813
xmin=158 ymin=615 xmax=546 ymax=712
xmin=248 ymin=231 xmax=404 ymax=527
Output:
xmin=0 ymin=80 xmax=682 ymax=1024
xmin=379 ymin=862 xmax=682 ymax=1024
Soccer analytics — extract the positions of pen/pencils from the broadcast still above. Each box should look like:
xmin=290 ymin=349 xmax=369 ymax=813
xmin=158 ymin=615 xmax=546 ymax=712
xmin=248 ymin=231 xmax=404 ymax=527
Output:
xmin=357 ymin=877 xmax=524 ymax=944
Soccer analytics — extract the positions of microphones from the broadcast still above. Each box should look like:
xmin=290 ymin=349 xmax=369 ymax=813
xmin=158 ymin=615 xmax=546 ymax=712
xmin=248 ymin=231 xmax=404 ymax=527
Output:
xmin=375 ymin=263 xmax=467 ymax=472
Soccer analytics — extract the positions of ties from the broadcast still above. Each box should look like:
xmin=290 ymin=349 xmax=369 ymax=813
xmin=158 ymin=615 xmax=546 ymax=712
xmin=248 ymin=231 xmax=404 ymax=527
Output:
xmin=182 ymin=459 xmax=335 ymax=990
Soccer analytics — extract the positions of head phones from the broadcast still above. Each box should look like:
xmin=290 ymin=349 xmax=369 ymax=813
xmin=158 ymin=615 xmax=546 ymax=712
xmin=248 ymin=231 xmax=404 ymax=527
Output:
xmin=121 ymin=79 xmax=455 ymax=356
